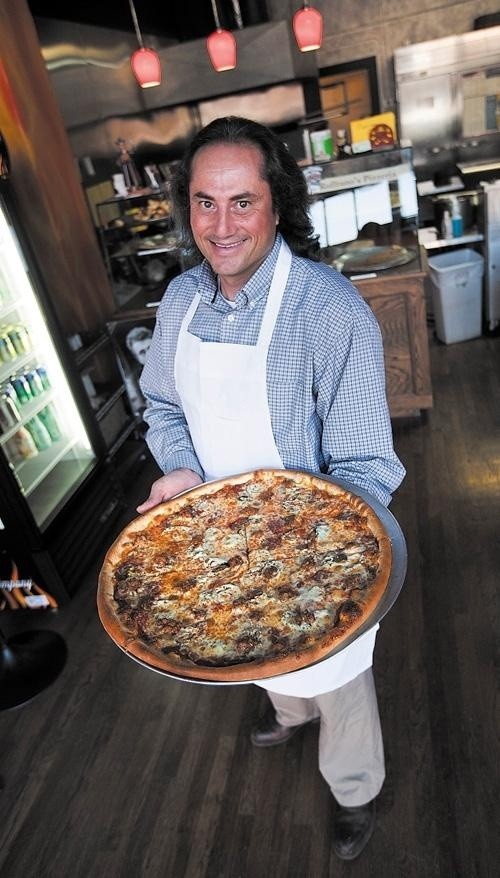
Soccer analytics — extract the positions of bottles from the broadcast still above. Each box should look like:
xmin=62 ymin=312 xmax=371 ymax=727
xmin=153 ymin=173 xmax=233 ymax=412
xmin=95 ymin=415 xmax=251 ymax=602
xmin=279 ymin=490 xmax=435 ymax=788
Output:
xmin=144 ymin=165 xmax=163 ymax=189
xmin=13 ymin=407 xmax=62 ymax=461
xmin=441 ymin=211 xmax=453 ymax=239
xmin=336 ymin=129 xmax=349 ymax=158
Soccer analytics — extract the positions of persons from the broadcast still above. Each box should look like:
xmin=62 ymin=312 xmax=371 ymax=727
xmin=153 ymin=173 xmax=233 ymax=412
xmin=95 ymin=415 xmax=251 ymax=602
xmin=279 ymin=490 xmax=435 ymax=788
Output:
xmin=134 ymin=111 xmax=409 ymax=863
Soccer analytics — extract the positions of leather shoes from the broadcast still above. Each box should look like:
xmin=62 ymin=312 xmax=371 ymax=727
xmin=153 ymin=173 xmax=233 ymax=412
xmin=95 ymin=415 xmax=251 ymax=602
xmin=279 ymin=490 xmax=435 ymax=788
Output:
xmin=331 ymin=799 xmax=375 ymax=861
xmin=250 ymin=704 xmax=320 ymax=747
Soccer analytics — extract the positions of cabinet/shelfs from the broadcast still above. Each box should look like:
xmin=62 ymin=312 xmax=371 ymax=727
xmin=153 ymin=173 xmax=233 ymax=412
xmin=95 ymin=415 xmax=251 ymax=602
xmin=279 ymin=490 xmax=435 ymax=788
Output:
xmin=94 ymin=193 xmax=184 ymax=312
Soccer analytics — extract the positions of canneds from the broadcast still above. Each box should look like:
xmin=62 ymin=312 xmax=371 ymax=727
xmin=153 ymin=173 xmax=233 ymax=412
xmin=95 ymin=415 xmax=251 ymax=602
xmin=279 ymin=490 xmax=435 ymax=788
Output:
xmin=2 ymin=322 xmax=62 ymax=462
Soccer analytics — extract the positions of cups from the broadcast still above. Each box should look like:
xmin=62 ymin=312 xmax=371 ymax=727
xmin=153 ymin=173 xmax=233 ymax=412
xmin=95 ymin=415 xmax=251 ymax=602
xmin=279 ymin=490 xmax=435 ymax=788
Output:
xmin=112 ymin=173 xmax=128 ymax=196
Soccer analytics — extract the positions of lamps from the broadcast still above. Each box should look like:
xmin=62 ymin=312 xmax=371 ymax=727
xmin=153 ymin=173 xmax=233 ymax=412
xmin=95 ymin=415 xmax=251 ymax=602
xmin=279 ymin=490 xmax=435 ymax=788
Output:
xmin=129 ymin=0 xmax=325 ymax=89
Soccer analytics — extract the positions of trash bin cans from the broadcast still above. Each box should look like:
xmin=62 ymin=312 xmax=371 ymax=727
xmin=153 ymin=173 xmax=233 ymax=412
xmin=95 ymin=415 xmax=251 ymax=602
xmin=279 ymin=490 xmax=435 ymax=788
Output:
xmin=429 ymin=247 xmax=487 ymax=346
